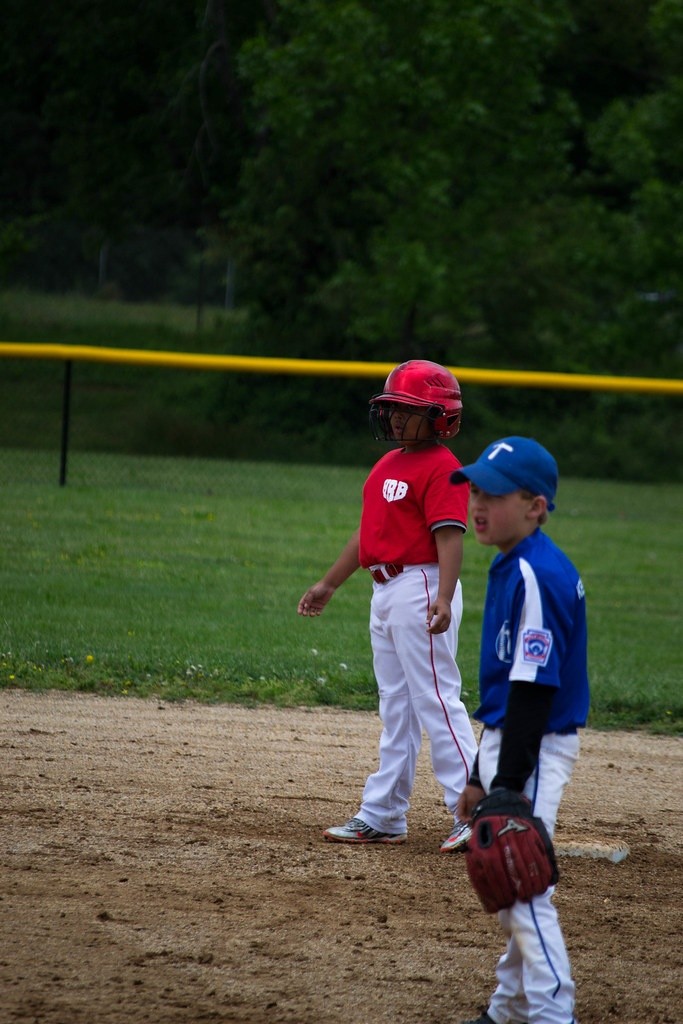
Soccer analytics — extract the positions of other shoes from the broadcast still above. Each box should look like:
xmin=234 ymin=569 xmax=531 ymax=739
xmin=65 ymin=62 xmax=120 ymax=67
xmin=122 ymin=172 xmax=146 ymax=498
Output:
xmin=460 ymin=1011 xmax=496 ymax=1024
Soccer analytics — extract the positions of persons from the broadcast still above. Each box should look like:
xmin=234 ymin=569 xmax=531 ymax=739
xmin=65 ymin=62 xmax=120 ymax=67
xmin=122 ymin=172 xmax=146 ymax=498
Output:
xmin=297 ymin=360 xmax=480 ymax=853
xmin=447 ymin=437 xmax=590 ymax=1024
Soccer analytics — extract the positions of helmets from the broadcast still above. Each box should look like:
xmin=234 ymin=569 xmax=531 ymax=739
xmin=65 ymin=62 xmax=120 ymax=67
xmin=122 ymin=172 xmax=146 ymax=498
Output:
xmin=369 ymin=359 xmax=463 ymax=440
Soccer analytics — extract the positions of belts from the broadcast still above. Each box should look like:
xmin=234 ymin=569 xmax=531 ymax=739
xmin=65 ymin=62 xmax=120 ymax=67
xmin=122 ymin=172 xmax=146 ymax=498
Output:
xmin=370 ymin=561 xmax=404 ymax=584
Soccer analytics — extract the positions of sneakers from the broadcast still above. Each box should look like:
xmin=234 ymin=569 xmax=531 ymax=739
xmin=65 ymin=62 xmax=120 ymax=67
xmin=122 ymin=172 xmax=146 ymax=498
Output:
xmin=323 ymin=817 xmax=408 ymax=843
xmin=440 ymin=818 xmax=473 ymax=851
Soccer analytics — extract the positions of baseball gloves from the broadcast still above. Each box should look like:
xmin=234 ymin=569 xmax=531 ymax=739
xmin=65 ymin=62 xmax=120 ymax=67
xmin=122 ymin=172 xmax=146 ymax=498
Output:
xmin=464 ymin=785 xmax=556 ymax=918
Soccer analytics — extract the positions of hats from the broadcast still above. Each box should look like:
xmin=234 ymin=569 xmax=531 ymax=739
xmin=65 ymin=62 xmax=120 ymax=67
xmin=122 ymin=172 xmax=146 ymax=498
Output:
xmin=449 ymin=437 xmax=559 ymax=512
